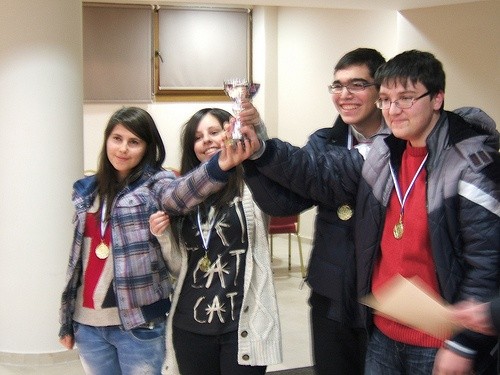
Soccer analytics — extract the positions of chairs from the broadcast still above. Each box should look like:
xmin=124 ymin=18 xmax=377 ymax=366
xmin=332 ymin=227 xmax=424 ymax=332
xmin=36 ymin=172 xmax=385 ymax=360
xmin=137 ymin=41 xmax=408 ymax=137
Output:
xmin=269 ymin=213 xmax=305 ymax=279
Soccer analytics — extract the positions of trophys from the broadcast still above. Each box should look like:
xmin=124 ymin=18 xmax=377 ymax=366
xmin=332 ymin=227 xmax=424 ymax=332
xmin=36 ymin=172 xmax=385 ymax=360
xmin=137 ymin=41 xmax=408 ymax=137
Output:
xmin=223 ymin=81 xmax=261 ymax=150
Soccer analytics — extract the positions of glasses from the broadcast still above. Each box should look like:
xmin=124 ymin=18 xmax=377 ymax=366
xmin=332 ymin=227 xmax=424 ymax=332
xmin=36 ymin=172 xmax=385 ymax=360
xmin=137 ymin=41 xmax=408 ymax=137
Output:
xmin=374 ymin=91 xmax=430 ymax=109
xmin=328 ymin=81 xmax=376 ymax=95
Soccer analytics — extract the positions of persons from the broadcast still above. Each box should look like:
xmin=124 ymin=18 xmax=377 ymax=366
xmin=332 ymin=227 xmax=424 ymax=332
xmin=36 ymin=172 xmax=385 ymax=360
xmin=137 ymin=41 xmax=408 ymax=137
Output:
xmin=58 ymin=109 xmax=262 ymax=375
xmin=145 ymin=102 xmax=284 ymax=375
xmin=223 ymin=48 xmax=500 ymax=375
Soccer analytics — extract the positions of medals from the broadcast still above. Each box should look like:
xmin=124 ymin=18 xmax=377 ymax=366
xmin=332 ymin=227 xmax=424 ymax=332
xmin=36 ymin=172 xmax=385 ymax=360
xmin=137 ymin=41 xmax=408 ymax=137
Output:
xmin=199 ymin=252 xmax=210 ymax=272
xmin=95 ymin=239 xmax=109 ymax=259
xmin=393 ymin=213 xmax=404 ymax=239
xmin=335 ymin=204 xmax=352 ymax=221
